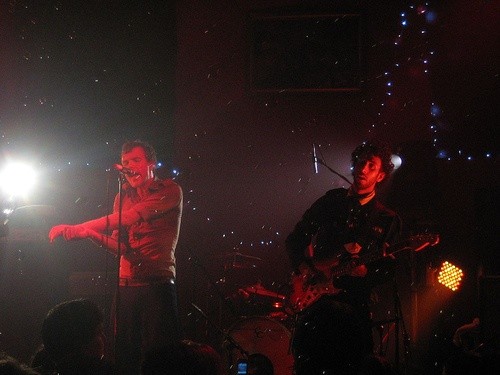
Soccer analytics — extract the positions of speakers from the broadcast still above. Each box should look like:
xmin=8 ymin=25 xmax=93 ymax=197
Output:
xmin=68 ymin=272 xmax=118 ymax=334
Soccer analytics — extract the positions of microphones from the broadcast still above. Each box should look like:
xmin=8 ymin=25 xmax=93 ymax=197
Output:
xmin=312 ymin=144 xmax=319 ymax=174
xmin=114 ymin=164 xmax=135 ymax=177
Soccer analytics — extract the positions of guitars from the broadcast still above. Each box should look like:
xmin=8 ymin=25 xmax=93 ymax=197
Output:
xmin=285 ymin=232 xmax=439 ymax=314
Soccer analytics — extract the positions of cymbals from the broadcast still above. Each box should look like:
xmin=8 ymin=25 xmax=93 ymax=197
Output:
xmin=243 ymin=285 xmax=285 ymax=300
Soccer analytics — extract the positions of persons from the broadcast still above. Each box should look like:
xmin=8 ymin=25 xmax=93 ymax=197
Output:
xmin=50 ymin=140 xmax=183 ymax=375
xmin=285 ymin=139 xmax=402 ymax=375
xmin=0 ymin=295 xmax=500 ymax=375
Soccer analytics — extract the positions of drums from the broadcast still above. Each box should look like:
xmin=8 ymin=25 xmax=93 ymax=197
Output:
xmin=222 ymin=316 xmax=295 ymax=375
xmin=264 ymin=304 xmax=287 ymax=321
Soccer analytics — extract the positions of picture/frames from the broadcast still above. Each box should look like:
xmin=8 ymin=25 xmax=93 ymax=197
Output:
xmin=244 ymin=8 xmax=366 ymax=96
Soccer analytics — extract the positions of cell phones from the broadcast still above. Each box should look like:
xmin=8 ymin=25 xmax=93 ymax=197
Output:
xmin=236 ymin=360 xmax=247 ymax=375
